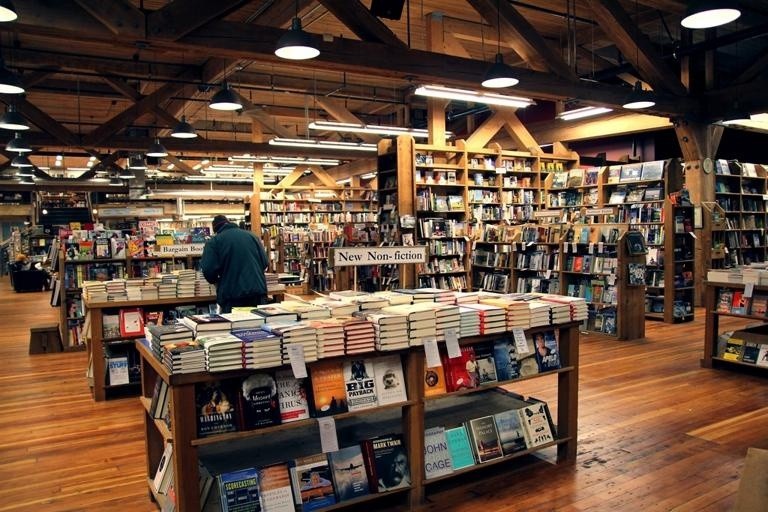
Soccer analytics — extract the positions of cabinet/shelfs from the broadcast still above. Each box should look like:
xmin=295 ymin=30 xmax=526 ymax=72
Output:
xmin=711 ymin=159 xmax=768 ymax=270
xmin=468 ymin=242 xmax=511 ymax=294
xmin=59 ymin=250 xmax=218 ymax=355
xmin=562 ymin=229 xmax=646 ymax=341
xmin=256 ymin=186 xmax=377 ymax=272
xmin=544 ymin=156 xmax=695 ymax=325
xmin=82 ymin=289 xmax=286 ymax=402
xmin=377 ymin=134 xmax=580 ymax=291
xmin=419 ymin=319 xmax=584 ymax=508
xmin=307 ymin=224 xmax=375 ymax=289
xmin=512 ymin=242 xmax=561 ymax=296
xmin=699 ymin=279 xmax=768 ymax=375
xmin=135 ymin=331 xmax=419 ymax=512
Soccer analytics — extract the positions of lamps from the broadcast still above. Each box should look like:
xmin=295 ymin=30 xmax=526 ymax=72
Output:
xmin=208 ymin=57 xmax=244 ymax=111
xmin=413 ymin=83 xmax=537 ymax=110
xmin=555 ymin=106 xmax=613 ymax=127
xmin=15 ymin=167 xmax=35 ymax=177
xmin=482 ymin=8 xmax=520 ymax=91
xmin=5 ymin=130 xmax=32 ymax=154
xmin=268 ymin=124 xmax=379 ymax=153
xmin=169 ymin=88 xmax=197 ymax=140
xmin=680 ymin=0 xmax=742 ymax=30
xmin=0 ymin=96 xmax=30 ymax=131
xmin=0 ymin=38 xmax=25 ymax=95
xmin=623 ymin=10 xmax=656 ymax=110
xmin=274 ymin=0 xmax=321 ymax=61
xmin=11 ymin=152 xmax=33 ymax=168
xmin=144 ymin=102 xmax=169 ymax=157
xmin=119 ymin=154 xmax=135 ymax=179
xmin=309 ymin=97 xmax=453 ymax=140
xmin=0 ymin=6 xmax=19 ymax=23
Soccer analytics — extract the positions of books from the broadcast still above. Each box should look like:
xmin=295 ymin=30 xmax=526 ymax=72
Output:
xmin=153 ymin=442 xmax=174 ymax=495
xmin=513 ymin=224 xmax=561 ymax=294
xmin=716 ymin=335 xmax=727 ymax=358
xmin=502 ymin=155 xmax=538 ymax=224
xmin=257 ymin=461 xmax=295 ymax=512
xmin=140 ymin=289 xmax=283 ymax=375
xmin=258 ymin=187 xmax=377 ymax=290
xmin=57 ymin=221 xmax=212 ymax=289
xmin=443 ymin=347 xmax=479 ymax=393
xmin=723 ymin=338 xmax=745 ymax=362
xmin=468 ymin=226 xmax=512 ymax=295
xmin=375 ymin=286 xmax=459 ymax=352
xmin=377 ymin=192 xmax=414 ymax=290
xmin=108 ymin=356 xmax=129 ymax=386
xmin=65 ymin=289 xmax=85 ymax=347
xmin=467 ymin=151 xmax=501 ymax=226
xmin=455 ymin=286 xmax=529 ymax=339
xmin=756 ymin=344 xmax=768 ymax=367
xmin=423 ymin=401 xmax=554 ymax=482
xmin=706 ymin=158 xmax=768 ymax=285
xmin=493 ymin=337 xmax=536 ymax=384
xmin=282 ymin=289 xmax=375 ymax=366
xmin=343 ymin=357 xmax=378 ymax=414
xmin=214 ymin=467 xmax=261 ymax=512
xmin=751 ymin=294 xmax=767 ymax=318
xmin=102 ymin=314 xmax=120 ymax=341
xmin=80 ymin=268 xmax=287 ymax=305
xmin=149 ymin=376 xmax=167 ymax=420
xmin=327 ymin=444 xmax=369 ymax=504
xmin=630 ymin=223 xmax=665 ymax=315
xmin=477 ymin=353 xmax=499 ymax=386
xmin=532 ymin=330 xmax=562 ymax=375
xmin=273 ymin=368 xmax=310 ymax=423
xmin=359 ymin=433 xmax=411 ymax=493
xmin=672 ymin=209 xmax=694 ymax=289
xmin=305 ymin=362 xmax=349 ymax=418
xmin=195 ymin=381 xmax=238 ymax=438
xmin=743 ymin=342 xmax=759 ymax=365
xmin=731 ymin=290 xmax=751 ymax=316
xmin=539 ymin=162 xmax=601 ymax=224
xmin=161 ymin=478 xmax=176 ymax=512
xmin=161 ymin=386 xmax=169 ymax=418
xmin=424 ymin=355 xmax=446 ymax=399
xmin=119 ymin=307 xmax=143 ymax=340
xmin=604 ymin=160 xmax=665 ymax=224
xmin=563 ymin=224 xmax=623 ymax=337
xmin=414 ymin=152 xmax=466 ymax=287
xmin=287 ymin=452 xmax=337 ymax=512
xmin=375 ymin=354 xmax=408 ymax=407
xmin=528 ymin=292 xmax=590 ymax=327
xmin=238 ymin=372 xmax=281 ymax=431
xmin=715 ymin=289 xmax=733 ymax=315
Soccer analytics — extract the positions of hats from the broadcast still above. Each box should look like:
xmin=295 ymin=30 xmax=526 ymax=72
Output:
xmin=211 ymin=214 xmax=228 ymax=232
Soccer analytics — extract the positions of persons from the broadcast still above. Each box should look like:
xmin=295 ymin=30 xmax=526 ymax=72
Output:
xmin=535 ymin=332 xmax=557 ymax=373
xmin=199 ymin=214 xmax=268 ymax=315
xmin=507 ymin=343 xmax=521 ymax=377
xmin=379 ymin=443 xmax=411 ymax=492
xmin=300 ymin=471 xmax=331 ymax=492
xmin=465 ymin=353 xmax=478 ymax=386
